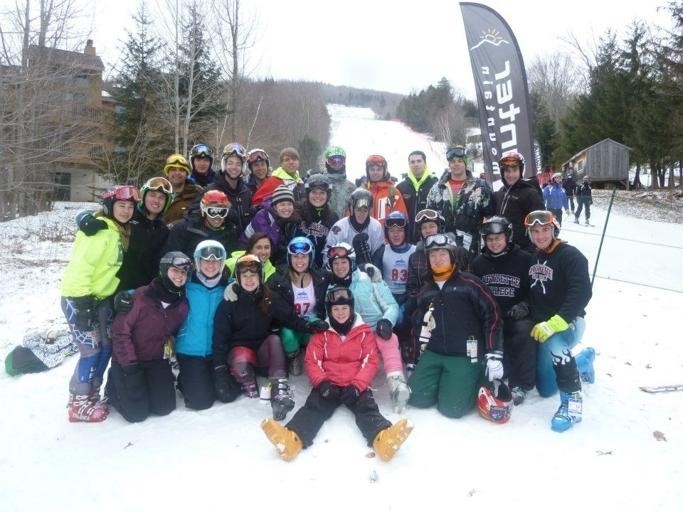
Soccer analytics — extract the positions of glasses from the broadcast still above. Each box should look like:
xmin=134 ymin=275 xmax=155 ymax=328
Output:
xmin=328 ymin=287 xmax=354 ymax=304
xmin=285 ymin=241 xmax=311 ymax=255
xmin=413 ymin=209 xmax=438 ymax=224
xmin=191 ymin=145 xmax=212 ymax=156
xmin=424 ymin=234 xmax=447 ymax=248
xmin=198 ymin=246 xmax=224 ymax=261
xmin=523 ymin=211 xmax=554 ymax=228
xmin=205 ymin=205 xmax=229 ymax=218
xmin=498 ymin=156 xmax=521 ymax=169
xmin=384 ymin=216 xmax=406 ymax=228
xmin=327 ymin=247 xmax=348 ymax=258
xmin=166 ymin=155 xmax=186 ymax=165
xmin=480 ymin=221 xmax=507 ymax=234
xmin=145 ymin=176 xmax=173 ymax=194
xmin=237 ymin=260 xmax=262 ymax=273
xmin=171 ymin=257 xmax=191 ymax=271
xmin=444 ymin=147 xmax=465 ymax=159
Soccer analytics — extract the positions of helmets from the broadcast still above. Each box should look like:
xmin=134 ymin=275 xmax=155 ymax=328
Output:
xmin=326 ymin=287 xmax=356 ymax=327
xmin=497 ymin=152 xmax=527 ymax=180
xmin=219 ymin=142 xmax=273 ymax=180
xmin=479 ymin=217 xmax=514 ymax=248
xmin=327 ymin=241 xmax=356 ymax=273
xmin=192 ymin=239 xmax=226 ymax=274
xmin=285 ymin=236 xmax=315 ymax=270
xmin=163 ymin=154 xmax=191 ymax=177
xmin=190 ymin=144 xmax=213 ymax=170
xmin=475 ymin=377 xmax=515 ymax=425
xmin=233 ymin=254 xmax=264 ymax=287
xmin=382 ymin=209 xmax=450 ymax=245
xmin=156 ymin=250 xmax=192 ymax=292
xmin=347 ymin=188 xmax=374 ymax=217
xmin=102 ymin=176 xmax=176 ymax=220
xmin=198 ymin=189 xmax=230 ymax=218
xmin=323 ymin=145 xmax=389 ymax=179
xmin=523 ymin=210 xmax=559 ymax=240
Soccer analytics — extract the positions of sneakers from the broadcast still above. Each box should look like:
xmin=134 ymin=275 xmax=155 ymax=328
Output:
xmin=259 ymin=416 xmax=303 ymax=464
xmin=511 ymin=384 xmax=526 ymax=407
xmin=269 ymin=377 xmax=297 ymax=421
xmin=573 ymin=219 xmax=579 ymax=225
xmin=370 ymin=415 xmax=417 ymax=462
xmin=67 ymin=394 xmax=111 ymax=423
xmin=584 ymin=219 xmax=590 ymax=225
xmin=549 ymin=390 xmax=585 ymax=432
xmin=574 ymin=345 xmax=597 ymax=385
xmin=386 ymin=374 xmax=411 ymax=414
xmin=240 ymin=378 xmax=260 ymax=398
xmin=289 ymin=354 xmax=303 ymax=376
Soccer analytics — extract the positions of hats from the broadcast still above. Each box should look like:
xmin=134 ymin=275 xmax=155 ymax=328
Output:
xmin=423 ymin=231 xmax=460 ymax=251
xmin=448 ymin=144 xmax=468 ymax=169
xmin=582 ymin=175 xmax=589 ymax=182
xmin=303 ymin=172 xmax=333 ymax=192
xmin=269 ymin=184 xmax=295 ymax=207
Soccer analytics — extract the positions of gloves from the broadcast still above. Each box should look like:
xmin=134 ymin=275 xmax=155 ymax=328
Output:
xmin=338 ymin=383 xmax=361 ymax=409
xmin=212 ymin=363 xmax=239 ymax=405
xmin=316 ymin=378 xmax=339 ymax=403
xmin=358 ymin=261 xmax=382 ymax=284
xmin=112 ymin=290 xmax=135 ymax=314
xmin=76 ymin=213 xmax=110 ymax=237
xmin=504 ymin=300 xmax=530 ymax=320
xmin=529 ymin=314 xmax=570 ymax=345
xmin=375 ymin=318 xmax=394 ymax=342
xmin=304 ymin=317 xmax=329 ymax=334
xmin=483 ymin=352 xmax=506 ymax=382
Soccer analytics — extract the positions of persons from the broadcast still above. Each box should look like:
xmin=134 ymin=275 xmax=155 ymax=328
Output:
xmin=61 ymin=143 xmax=595 ymax=461
